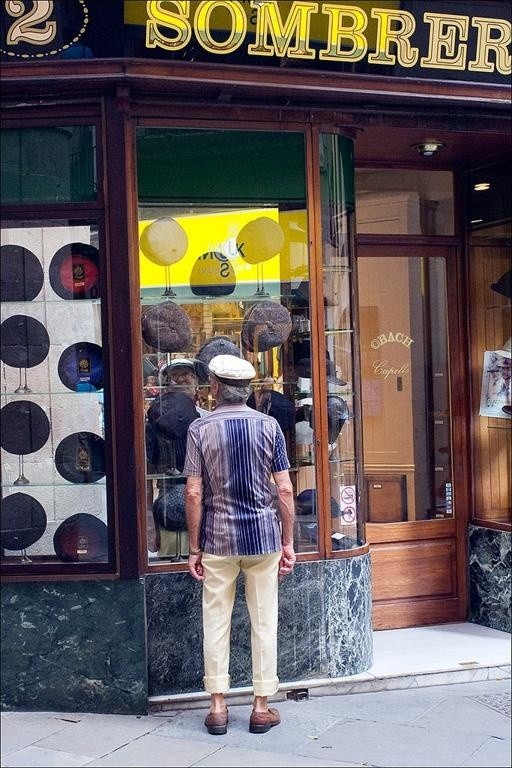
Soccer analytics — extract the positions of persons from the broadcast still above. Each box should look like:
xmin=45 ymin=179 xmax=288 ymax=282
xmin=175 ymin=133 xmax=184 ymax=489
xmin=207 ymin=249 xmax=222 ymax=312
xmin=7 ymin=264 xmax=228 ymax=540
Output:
xmin=486 ymin=358 xmax=511 ymax=407
xmin=144 ymin=360 xmax=207 ymax=554
xmin=184 ymin=353 xmax=297 ymax=734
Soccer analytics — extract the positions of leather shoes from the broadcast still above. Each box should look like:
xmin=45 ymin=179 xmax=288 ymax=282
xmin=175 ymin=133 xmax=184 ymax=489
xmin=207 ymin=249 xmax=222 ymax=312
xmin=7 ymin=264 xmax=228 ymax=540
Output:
xmin=249 ymin=708 xmax=281 ymax=732
xmin=205 ymin=706 xmax=228 ymax=734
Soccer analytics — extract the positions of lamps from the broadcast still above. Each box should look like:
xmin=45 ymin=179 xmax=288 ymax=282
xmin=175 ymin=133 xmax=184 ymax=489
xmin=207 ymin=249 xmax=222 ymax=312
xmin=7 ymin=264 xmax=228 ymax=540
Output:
xmin=410 ymin=139 xmax=445 ymax=156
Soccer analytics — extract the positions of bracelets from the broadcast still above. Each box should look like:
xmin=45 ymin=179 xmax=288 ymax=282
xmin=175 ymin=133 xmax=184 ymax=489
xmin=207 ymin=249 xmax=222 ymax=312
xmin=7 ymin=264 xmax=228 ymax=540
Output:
xmin=188 ymin=545 xmax=201 ymax=555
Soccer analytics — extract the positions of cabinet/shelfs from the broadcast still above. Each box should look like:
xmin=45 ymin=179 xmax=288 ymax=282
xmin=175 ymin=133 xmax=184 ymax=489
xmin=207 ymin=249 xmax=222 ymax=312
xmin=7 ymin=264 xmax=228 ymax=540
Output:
xmin=139 ymin=293 xmax=357 ymax=574
xmin=0 ymin=299 xmax=117 ymax=576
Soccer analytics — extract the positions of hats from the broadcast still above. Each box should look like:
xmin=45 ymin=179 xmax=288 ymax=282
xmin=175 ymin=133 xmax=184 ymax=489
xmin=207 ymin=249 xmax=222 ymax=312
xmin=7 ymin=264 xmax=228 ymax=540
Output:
xmin=491 ymin=269 xmax=512 ymax=298
xmin=238 ymin=215 xmax=285 ymax=264
xmin=55 ymin=431 xmax=106 ymax=484
xmin=141 ymin=302 xmax=192 ymax=354
xmin=0 ymin=245 xmax=45 ymax=302
xmin=54 ymin=513 xmax=109 ymax=563
xmin=495 ymin=337 xmax=512 ymax=358
xmin=1 ymin=492 xmax=47 ymax=551
xmin=242 ymin=300 xmax=293 ymax=354
xmin=49 ymin=243 xmax=100 ymax=300
xmin=139 ymin=217 xmax=187 ymax=267
xmin=193 ymin=336 xmax=257 ymax=386
xmin=279 ymin=211 xmax=355 ymax=519
xmin=498 ymin=359 xmax=512 ymax=367
xmin=58 ymin=342 xmax=105 ymax=393
xmin=1 ymin=401 xmax=50 ymax=455
xmin=1 ymin=315 xmax=50 ymax=368
xmin=191 ymin=252 xmax=237 ymax=296
xmin=144 ymin=390 xmax=201 ymax=533
xmin=246 ymin=390 xmax=296 ymax=434
xmin=158 ymin=359 xmax=207 ymax=391
